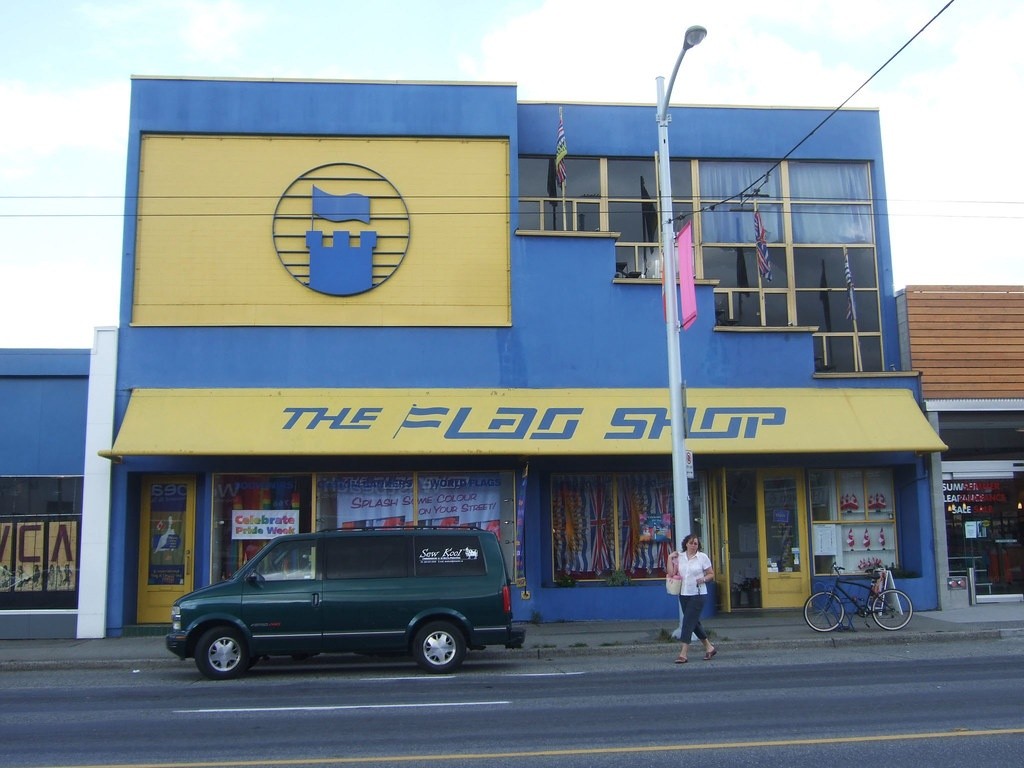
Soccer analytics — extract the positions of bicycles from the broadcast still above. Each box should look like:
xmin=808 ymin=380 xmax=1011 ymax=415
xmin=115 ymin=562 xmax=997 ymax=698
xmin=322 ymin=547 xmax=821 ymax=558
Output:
xmin=804 ymin=561 xmax=913 ymax=634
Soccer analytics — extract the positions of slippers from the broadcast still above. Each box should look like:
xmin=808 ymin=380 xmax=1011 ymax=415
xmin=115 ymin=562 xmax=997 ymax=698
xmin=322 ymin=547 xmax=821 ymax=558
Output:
xmin=674 ymin=657 xmax=688 ymax=663
xmin=703 ymin=649 xmax=717 ymax=660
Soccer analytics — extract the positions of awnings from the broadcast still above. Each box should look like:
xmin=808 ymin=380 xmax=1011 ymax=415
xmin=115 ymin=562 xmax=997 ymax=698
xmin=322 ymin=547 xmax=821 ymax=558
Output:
xmin=98 ymin=385 xmax=950 ymax=458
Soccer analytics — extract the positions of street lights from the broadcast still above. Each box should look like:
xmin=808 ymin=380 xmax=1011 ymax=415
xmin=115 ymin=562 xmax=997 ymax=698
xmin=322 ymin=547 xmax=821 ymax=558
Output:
xmin=656 ymin=25 xmax=708 ymax=641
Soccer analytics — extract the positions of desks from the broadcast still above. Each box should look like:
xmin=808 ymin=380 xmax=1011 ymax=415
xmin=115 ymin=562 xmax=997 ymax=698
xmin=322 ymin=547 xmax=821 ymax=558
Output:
xmin=979 ymin=538 xmax=1018 ymax=592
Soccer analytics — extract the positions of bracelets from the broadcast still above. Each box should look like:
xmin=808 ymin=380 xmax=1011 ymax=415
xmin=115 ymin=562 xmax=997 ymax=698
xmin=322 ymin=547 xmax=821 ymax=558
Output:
xmin=703 ymin=578 xmax=706 ymax=582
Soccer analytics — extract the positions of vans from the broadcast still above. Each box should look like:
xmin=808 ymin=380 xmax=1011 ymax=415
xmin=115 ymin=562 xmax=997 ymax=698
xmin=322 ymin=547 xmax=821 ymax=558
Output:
xmin=166 ymin=524 xmax=526 ymax=681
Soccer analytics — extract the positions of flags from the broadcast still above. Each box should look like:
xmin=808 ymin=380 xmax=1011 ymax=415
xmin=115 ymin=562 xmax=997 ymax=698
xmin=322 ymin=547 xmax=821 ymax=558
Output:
xmin=844 ymin=253 xmax=856 ymax=321
xmin=753 ymin=209 xmax=774 ymax=284
xmin=556 ymin=116 xmax=568 ymax=191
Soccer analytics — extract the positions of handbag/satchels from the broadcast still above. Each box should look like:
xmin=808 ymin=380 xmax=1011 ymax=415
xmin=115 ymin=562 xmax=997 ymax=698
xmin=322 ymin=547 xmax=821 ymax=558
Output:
xmin=666 ymin=562 xmax=683 ymax=595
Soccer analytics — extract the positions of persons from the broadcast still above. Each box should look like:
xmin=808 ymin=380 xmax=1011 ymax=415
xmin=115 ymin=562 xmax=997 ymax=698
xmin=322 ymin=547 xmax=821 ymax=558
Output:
xmin=667 ymin=534 xmax=718 ymax=664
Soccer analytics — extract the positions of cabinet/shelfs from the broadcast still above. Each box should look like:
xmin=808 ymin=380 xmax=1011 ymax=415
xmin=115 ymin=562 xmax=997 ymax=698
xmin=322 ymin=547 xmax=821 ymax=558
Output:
xmin=841 ymin=509 xmax=895 ymax=552
xmin=948 ymin=556 xmax=993 ymax=595
